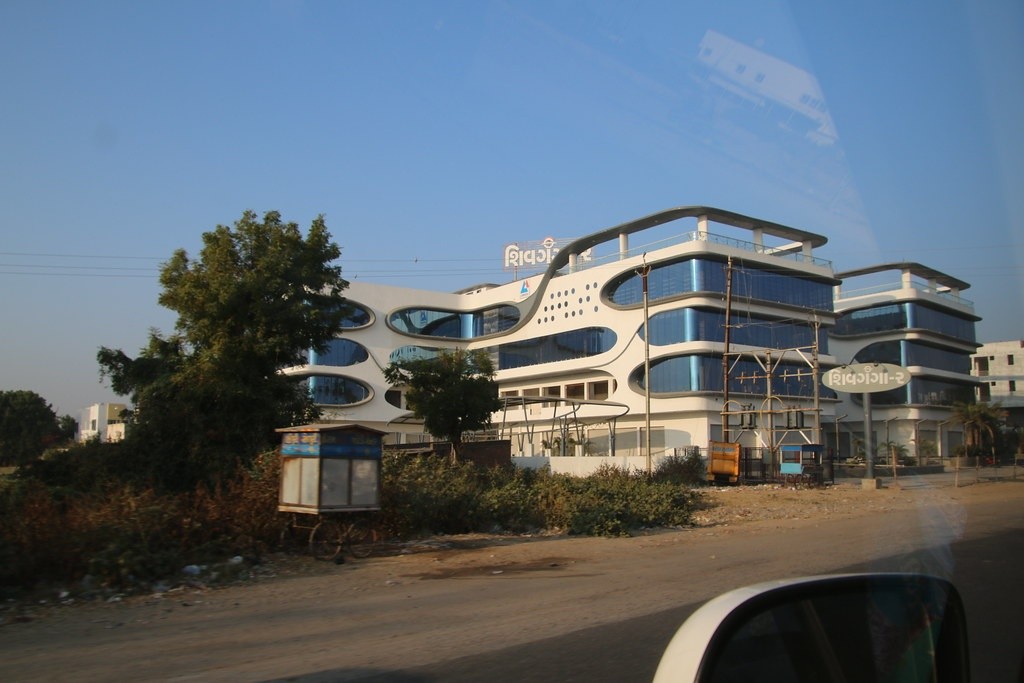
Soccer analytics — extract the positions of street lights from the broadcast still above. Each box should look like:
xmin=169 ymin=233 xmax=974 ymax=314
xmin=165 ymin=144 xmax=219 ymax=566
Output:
xmin=938 ymin=419 xmax=951 ymax=458
xmin=885 ymin=416 xmax=899 ymax=465
xmin=916 ymin=417 xmax=928 ymax=465
xmin=964 ymin=419 xmax=975 ymax=459
xmin=836 ymin=414 xmax=848 ymax=463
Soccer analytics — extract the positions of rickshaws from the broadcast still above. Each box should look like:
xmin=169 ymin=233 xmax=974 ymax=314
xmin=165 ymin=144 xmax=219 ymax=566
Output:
xmin=779 ymin=444 xmax=824 ymax=491
xmin=275 ymin=423 xmax=389 ymax=563
